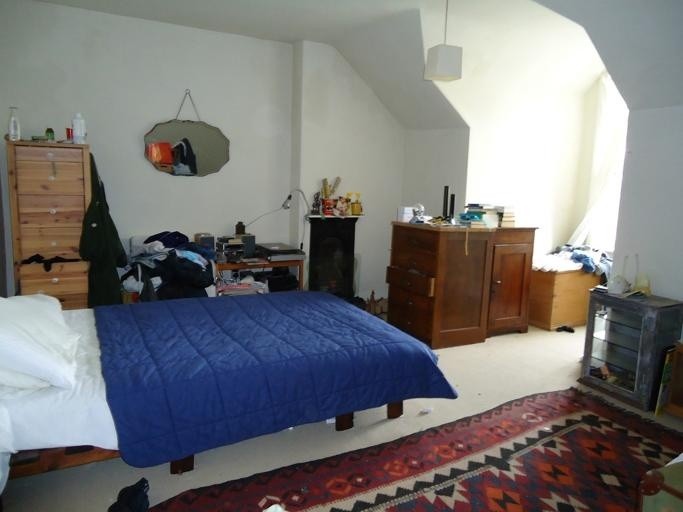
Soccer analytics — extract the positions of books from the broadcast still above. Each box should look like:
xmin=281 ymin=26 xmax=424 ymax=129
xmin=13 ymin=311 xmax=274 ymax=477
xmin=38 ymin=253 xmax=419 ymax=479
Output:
xmin=216 ymin=235 xmax=245 ymax=262
xmin=460 ymin=203 xmax=514 ymax=229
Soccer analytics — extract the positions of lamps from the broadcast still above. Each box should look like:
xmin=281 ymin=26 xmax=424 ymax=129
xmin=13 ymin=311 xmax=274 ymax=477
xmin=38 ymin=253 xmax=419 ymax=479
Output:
xmin=423 ymin=0 xmax=463 ymax=85
xmin=282 ymin=187 xmax=312 ymax=221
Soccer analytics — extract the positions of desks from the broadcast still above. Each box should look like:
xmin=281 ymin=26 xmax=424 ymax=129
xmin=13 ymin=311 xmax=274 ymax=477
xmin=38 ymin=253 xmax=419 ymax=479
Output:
xmin=211 ymin=254 xmax=304 ymax=294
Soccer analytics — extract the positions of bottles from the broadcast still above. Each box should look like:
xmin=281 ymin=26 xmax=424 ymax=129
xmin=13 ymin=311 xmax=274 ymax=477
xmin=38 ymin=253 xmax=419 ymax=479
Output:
xmin=71 ymin=113 xmax=86 ymax=145
xmin=234 ymin=222 xmax=245 ymax=233
xmin=45 ymin=128 xmax=53 ymax=141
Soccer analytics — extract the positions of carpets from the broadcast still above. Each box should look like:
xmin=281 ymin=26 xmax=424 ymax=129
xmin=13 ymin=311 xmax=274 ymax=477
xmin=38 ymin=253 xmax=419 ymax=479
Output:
xmin=150 ymin=378 xmax=683 ymax=510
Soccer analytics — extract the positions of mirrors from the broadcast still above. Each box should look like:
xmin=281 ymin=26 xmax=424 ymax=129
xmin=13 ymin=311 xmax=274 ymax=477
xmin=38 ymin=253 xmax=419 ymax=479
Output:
xmin=143 ymin=116 xmax=231 ymax=177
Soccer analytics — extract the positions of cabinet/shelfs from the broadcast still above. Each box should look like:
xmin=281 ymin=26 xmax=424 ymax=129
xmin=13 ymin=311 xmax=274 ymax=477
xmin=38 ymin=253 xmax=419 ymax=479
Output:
xmin=305 ymin=213 xmax=356 ymax=303
xmin=487 ymin=225 xmax=542 ymax=339
xmin=384 ymin=221 xmax=495 ymax=349
xmin=576 ymin=282 xmax=681 ymax=414
xmin=3 ymin=135 xmax=95 ymax=313
xmin=523 ymin=262 xmax=606 ymax=332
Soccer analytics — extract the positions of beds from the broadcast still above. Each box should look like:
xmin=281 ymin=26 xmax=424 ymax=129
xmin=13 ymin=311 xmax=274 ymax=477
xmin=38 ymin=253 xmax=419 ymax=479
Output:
xmin=0 ymin=287 xmax=461 ymax=488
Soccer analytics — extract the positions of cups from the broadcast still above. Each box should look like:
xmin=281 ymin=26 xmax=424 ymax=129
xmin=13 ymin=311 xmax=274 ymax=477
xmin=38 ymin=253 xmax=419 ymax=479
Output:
xmin=321 ymin=198 xmax=337 ymax=215
xmin=351 ymin=203 xmax=361 ymax=215
